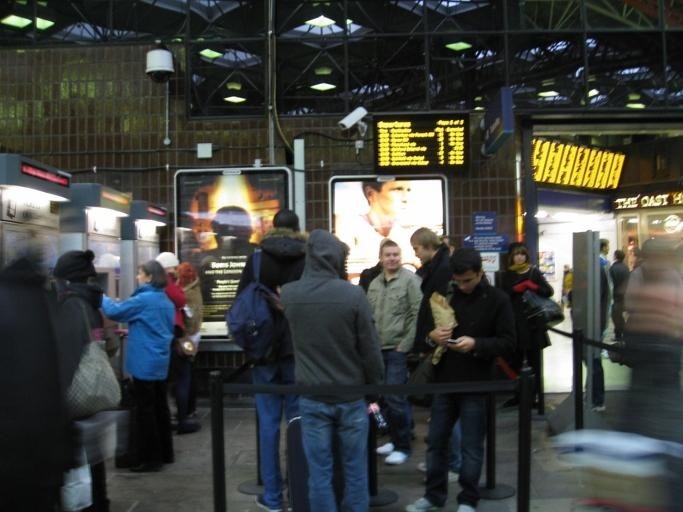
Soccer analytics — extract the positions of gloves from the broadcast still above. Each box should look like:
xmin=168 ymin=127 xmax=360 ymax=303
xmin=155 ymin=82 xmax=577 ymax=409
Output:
xmin=514 ymin=281 xmax=539 ymax=292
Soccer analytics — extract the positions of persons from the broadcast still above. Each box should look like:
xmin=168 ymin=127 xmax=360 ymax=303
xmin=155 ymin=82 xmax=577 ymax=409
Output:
xmin=563 ymin=266 xmax=574 ymax=309
xmin=503 ymin=242 xmax=554 ymax=408
xmin=409 ymin=228 xmax=462 ymax=483
xmin=272 ymin=230 xmax=386 ymax=512
xmin=342 ymin=179 xmax=424 ymax=285
xmin=235 ymin=210 xmax=299 ymax=296
xmin=367 ymin=240 xmax=422 ymax=463
xmin=51 ymin=250 xmax=110 ymax=512
xmin=228 ymin=237 xmax=302 ymax=511
xmin=566 ymin=275 xmax=612 ymax=410
xmin=599 ymin=239 xmax=612 ymax=274
xmin=172 ymin=261 xmax=203 ymax=435
xmin=610 ymin=252 xmax=629 ymax=342
xmin=156 ymin=251 xmax=186 ymax=393
xmin=102 ymin=261 xmax=175 ymax=474
xmin=358 ymin=238 xmax=392 ymax=291
xmin=403 ymin=247 xmax=515 ymax=511
xmin=624 ymin=236 xmax=682 ymax=438
xmin=2 ymin=238 xmax=92 ymax=511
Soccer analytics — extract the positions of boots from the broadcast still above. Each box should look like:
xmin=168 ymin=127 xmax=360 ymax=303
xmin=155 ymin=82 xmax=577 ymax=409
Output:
xmin=504 ymin=374 xmax=539 ymax=408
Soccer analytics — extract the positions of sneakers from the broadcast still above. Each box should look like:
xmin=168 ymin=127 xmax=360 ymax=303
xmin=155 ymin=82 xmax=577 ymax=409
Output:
xmin=376 ymin=442 xmax=408 ymax=464
xmin=456 ymin=504 xmax=475 ymax=512
xmin=417 ymin=461 xmax=460 ymax=484
xmin=406 ymin=498 xmax=441 ymax=512
xmin=255 ymin=494 xmax=292 ymax=511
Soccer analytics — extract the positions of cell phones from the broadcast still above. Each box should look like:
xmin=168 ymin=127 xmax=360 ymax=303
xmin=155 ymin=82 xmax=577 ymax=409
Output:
xmin=443 ymin=337 xmax=463 ymax=345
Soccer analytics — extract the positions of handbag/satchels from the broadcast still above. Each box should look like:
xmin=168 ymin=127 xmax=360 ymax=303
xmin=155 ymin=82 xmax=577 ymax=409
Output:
xmin=226 ymin=282 xmax=294 ymax=371
xmin=402 ymin=355 xmax=439 ymax=407
xmin=182 ymin=305 xmax=202 ymax=333
xmin=522 ymin=290 xmax=564 ymax=330
xmin=174 ymin=337 xmax=196 ymax=360
xmin=67 ymin=340 xmax=122 ymax=417
xmin=371 ymin=401 xmax=406 ymax=434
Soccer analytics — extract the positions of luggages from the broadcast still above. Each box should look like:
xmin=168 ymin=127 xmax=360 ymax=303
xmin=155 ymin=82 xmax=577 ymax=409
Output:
xmin=287 ymin=416 xmax=344 ymax=512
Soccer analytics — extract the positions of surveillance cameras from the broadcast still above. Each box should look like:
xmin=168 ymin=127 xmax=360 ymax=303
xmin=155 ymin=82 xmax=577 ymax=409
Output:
xmin=144 ymin=48 xmax=177 ymax=84
xmin=337 ymin=104 xmax=370 ymax=132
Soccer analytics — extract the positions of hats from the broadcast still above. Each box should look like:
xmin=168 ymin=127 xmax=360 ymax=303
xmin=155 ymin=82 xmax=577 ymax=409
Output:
xmin=54 ymin=251 xmax=96 ymax=281
xmin=176 ymin=263 xmax=197 ymax=286
xmin=509 ymin=242 xmax=528 ymax=253
xmin=156 ymin=251 xmax=180 ymax=269
